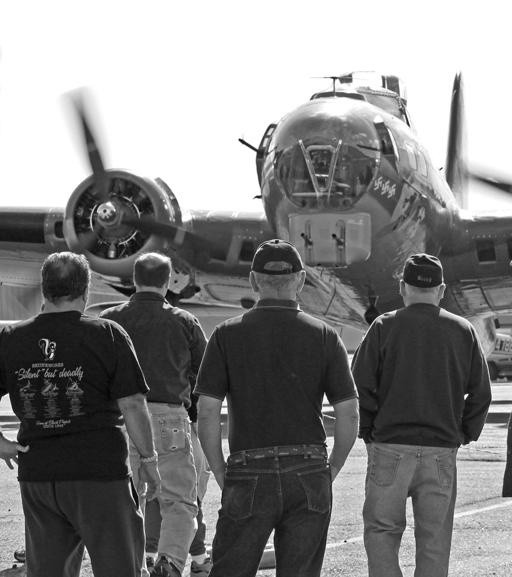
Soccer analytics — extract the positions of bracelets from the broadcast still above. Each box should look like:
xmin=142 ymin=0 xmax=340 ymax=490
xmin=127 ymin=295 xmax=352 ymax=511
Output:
xmin=138 ymin=450 xmax=158 ymax=466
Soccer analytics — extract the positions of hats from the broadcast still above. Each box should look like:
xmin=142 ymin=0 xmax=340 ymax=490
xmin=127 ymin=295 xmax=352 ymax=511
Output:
xmin=250 ymin=238 xmax=305 ymax=275
xmin=402 ymin=252 xmax=444 ymax=287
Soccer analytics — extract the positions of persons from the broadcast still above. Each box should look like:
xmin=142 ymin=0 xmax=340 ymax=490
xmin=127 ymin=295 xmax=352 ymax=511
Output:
xmin=0 ymin=251 xmax=162 ymax=576
xmin=190 ymin=238 xmax=362 ymax=576
xmin=99 ymin=251 xmax=207 ymax=577
xmin=351 ymin=253 xmax=493 ymax=576
xmin=12 ymin=546 xmax=25 ymax=565
xmin=144 ymin=288 xmax=213 ymax=576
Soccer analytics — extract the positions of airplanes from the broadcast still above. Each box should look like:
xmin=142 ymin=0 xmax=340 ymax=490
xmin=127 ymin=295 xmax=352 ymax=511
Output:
xmin=0 ymin=67 xmax=512 ymax=363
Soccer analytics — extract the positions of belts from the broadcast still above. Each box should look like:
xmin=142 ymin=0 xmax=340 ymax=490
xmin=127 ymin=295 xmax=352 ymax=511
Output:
xmin=228 ymin=443 xmax=328 ymax=464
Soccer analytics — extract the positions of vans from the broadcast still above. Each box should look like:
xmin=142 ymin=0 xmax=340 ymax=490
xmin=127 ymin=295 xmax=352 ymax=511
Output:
xmin=481 ymin=332 xmax=512 ymax=380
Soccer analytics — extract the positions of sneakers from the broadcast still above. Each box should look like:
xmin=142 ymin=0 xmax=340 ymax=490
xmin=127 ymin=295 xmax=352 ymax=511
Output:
xmin=14 ymin=549 xmax=26 ymax=563
xmin=144 ymin=552 xmax=213 ymax=576
